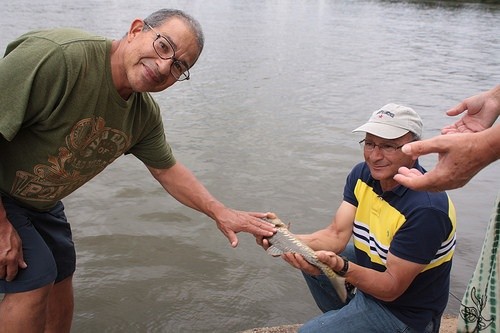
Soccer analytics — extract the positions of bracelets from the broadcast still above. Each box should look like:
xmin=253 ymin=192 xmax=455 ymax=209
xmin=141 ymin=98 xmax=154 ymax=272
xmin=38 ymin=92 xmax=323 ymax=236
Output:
xmin=332 ymin=254 xmax=349 ymax=278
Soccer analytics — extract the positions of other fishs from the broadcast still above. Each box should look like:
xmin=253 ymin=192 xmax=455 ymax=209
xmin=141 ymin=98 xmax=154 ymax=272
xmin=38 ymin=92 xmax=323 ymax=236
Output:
xmin=258 ymin=215 xmax=348 ymax=304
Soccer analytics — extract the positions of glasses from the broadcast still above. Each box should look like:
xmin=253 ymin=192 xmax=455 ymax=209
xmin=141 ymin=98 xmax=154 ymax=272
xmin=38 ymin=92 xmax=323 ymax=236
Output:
xmin=144 ymin=21 xmax=190 ymax=83
xmin=358 ymin=139 xmax=414 ymax=153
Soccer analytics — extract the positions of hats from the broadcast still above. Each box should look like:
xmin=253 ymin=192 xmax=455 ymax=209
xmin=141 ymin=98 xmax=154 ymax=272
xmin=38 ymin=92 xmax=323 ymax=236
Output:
xmin=352 ymin=103 xmax=423 ymax=139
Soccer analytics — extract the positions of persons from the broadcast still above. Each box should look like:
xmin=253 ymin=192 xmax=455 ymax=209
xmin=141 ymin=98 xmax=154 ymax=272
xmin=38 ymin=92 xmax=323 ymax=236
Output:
xmin=392 ymin=81 xmax=500 ymax=333
xmin=253 ymin=102 xmax=459 ymax=333
xmin=0 ymin=8 xmax=280 ymax=333
